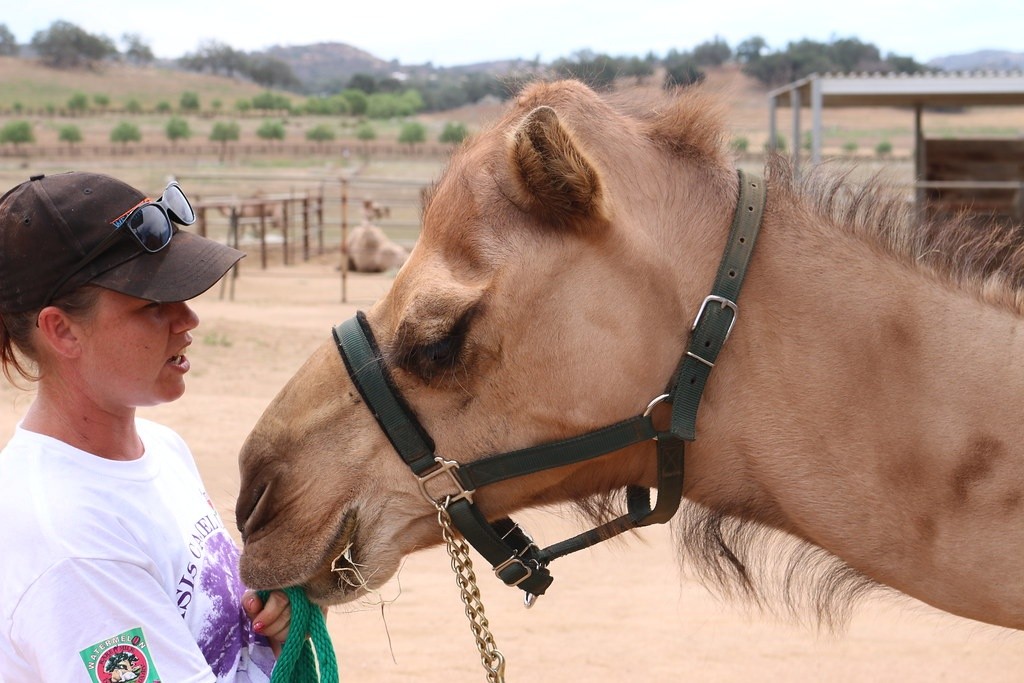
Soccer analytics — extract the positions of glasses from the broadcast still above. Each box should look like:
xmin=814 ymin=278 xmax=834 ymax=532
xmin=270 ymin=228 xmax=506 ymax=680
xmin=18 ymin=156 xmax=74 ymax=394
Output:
xmin=37 ymin=181 xmax=196 ymax=327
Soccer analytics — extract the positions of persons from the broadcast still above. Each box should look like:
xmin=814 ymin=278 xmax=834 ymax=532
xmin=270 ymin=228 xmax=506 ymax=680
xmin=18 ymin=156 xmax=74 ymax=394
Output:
xmin=0 ymin=168 xmax=327 ymax=683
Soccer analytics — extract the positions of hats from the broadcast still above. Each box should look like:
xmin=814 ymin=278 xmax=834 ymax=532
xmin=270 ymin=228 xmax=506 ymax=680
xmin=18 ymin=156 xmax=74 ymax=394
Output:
xmin=0 ymin=171 xmax=247 ymax=316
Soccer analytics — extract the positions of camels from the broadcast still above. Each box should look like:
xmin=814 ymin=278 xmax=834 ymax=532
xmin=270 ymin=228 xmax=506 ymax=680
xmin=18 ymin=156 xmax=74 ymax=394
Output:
xmin=234 ymin=78 xmax=1024 ymax=635
xmin=347 ymin=223 xmax=409 ymax=273
